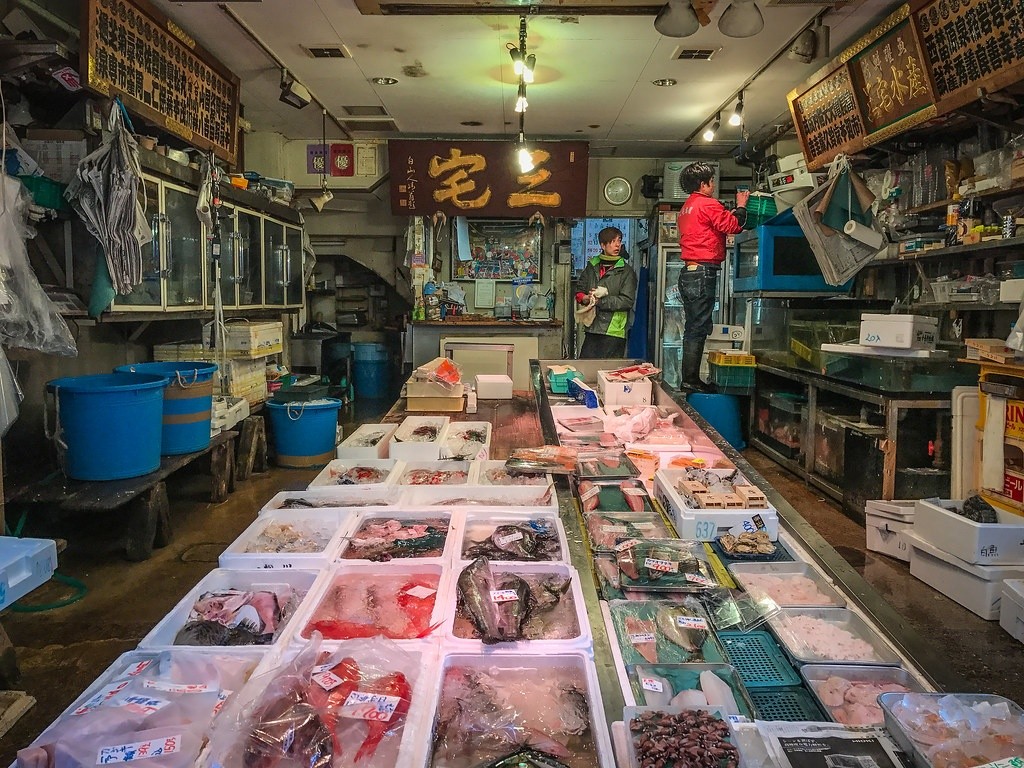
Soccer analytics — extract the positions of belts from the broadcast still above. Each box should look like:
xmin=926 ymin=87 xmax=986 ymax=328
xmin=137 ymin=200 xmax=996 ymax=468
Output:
xmin=685 ymin=266 xmax=722 ymax=271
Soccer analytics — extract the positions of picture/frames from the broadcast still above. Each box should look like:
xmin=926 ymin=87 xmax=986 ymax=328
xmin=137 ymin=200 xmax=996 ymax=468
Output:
xmin=449 ymin=216 xmax=543 ymax=284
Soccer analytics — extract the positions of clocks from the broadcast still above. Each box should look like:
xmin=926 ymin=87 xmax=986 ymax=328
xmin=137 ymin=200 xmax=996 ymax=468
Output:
xmin=603 ymin=176 xmax=632 ymax=206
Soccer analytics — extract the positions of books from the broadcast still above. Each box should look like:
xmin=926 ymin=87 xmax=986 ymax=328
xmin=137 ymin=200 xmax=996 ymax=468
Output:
xmin=965 ymin=337 xmax=1016 ymax=364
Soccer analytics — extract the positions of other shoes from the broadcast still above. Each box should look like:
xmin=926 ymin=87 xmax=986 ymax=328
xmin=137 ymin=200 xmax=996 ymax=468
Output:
xmin=680 ymin=378 xmax=717 ymax=394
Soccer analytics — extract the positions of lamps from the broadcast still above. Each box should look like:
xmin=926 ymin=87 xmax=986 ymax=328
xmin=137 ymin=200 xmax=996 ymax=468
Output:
xmin=515 ymin=84 xmax=528 ymax=113
xmin=278 ymin=67 xmax=311 ymax=109
xmin=654 ymin=0 xmax=699 ymax=38
xmin=524 ymin=54 xmax=536 ymax=82
xmin=309 ymin=109 xmax=334 ymax=214
xmin=703 ymin=111 xmax=721 ymax=141
xmin=514 ymin=137 xmax=534 ymax=174
xmin=506 ymin=43 xmax=526 ymax=75
xmin=719 ymin=0 xmax=764 ymax=38
xmin=729 ymin=91 xmax=743 ymax=126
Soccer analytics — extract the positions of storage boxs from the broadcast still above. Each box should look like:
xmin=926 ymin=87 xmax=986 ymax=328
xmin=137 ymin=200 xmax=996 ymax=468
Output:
xmin=737 ymin=195 xmax=776 ymax=229
xmin=864 ymin=499 xmax=1024 ymax=643
xmin=859 ymin=314 xmax=939 ymax=348
xmin=530 ymin=310 xmax=549 ymax=319
xmin=22 ymin=127 xmax=88 ymax=182
xmin=0 ymin=323 xmax=779 ymax=768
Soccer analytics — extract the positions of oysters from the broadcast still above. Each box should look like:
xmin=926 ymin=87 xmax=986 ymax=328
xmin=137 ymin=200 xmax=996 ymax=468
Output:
xmin=630 ymin=710 xmax=739 ymax=768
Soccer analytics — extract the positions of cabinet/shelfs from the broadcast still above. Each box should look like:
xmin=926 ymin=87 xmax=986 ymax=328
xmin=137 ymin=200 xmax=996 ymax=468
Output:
xmin=750 ymin=364 xmax=954 ymax=517
xmin=859 ymin=181 xmax=1024 ymax=312
xmin=335 ymin=283 xmax=368 ymax=325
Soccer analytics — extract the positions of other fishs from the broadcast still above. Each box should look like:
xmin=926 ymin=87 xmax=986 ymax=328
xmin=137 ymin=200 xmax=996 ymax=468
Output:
xmin=172 ymin=507 xmax=446 ymax=768
xmin=456 ymin=554 xmax=573 ymax=645
xmin=462 ymin=515 xmax=564 ymax=562
xmin=430 ymin=660 xmax=597 ymax=768
xmin=578 ymin=475 xmax=741 ymax=716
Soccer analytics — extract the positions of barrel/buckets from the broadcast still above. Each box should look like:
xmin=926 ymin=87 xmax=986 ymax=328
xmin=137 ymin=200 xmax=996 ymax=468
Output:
xmin=685 ymin=393 xmax=746 ymax=451
xmin=265 ymin=398 xmax=342 ymax=469
xmin=43 ymin=372 xmax=169 ymax=481
xmin=352 ymin=342 xmax=389 ymax=362
xmin=114 ymin=361 xmax=218 ymax=455
xmin=352 ymin=359 xmax=389 ymax=398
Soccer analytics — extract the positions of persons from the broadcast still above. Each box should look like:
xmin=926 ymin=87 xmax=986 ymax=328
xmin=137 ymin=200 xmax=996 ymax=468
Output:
xmin=575 ymin=227 xmax=638 ymax=360
xmin=678 ymin=162 xmax=750 ymax=393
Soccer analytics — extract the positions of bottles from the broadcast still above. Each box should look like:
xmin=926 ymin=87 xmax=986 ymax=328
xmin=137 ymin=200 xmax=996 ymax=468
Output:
xmin=947 ymin=194 xmax=960 ymax=226
